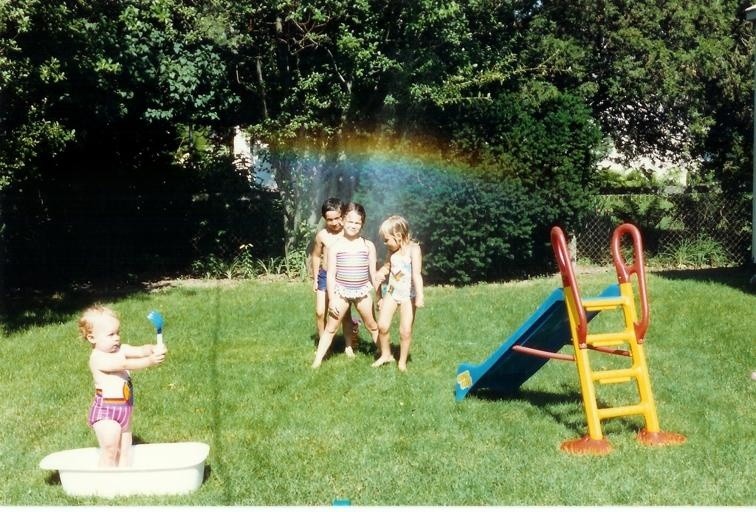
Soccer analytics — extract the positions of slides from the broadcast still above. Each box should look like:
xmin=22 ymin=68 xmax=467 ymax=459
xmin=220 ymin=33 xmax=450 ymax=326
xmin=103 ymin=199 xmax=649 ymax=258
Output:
xmin=456 ymin=284 xmax=622 ymax=400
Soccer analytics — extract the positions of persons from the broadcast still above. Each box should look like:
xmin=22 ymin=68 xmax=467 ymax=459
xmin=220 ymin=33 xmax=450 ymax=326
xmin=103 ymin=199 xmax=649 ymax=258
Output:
xmin=313 ymin=197 xmax=355 ymax=358
xmin=311 ymin=202 xmax=380 ymax=367
xmin=370 ymin=215 xmax=423 ymax=370
xmin=79 ymin=304 xmax=168 ymax=467
